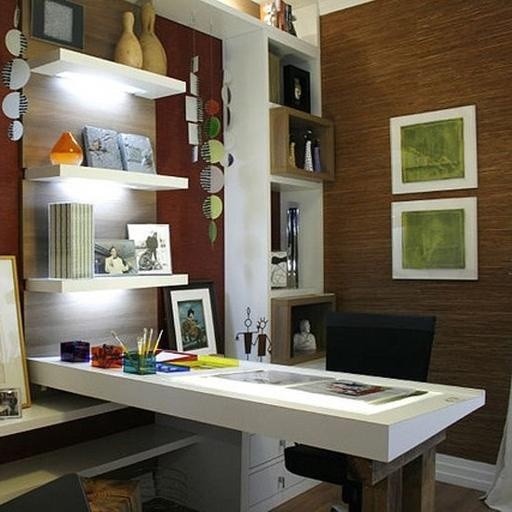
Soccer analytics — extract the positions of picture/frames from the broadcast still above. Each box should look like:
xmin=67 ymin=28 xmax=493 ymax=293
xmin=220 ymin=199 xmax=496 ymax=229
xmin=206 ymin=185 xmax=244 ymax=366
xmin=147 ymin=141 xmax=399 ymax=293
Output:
xmin=389 ymin=106 xmax=478 ymax=195
xmin=389 ymin=197 xmax=480 ymax=283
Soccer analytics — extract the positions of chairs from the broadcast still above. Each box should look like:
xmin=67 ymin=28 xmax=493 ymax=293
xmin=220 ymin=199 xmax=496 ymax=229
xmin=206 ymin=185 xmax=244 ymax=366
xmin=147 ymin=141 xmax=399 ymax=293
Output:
xmin=283 ymin=310 xmax=439 ymax=510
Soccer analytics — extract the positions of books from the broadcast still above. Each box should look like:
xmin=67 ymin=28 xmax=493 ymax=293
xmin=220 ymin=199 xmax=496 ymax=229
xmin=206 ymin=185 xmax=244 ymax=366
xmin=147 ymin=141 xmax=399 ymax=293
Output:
xmin=82 ymin=125 xmax=124 ymax=171
xmin=283 ymin=4 xmax=293 ymax=34
xmin=116 ymin=133 xmax=157 ymax=176
xmin=259 ymin=0 xmax=286 ymax=32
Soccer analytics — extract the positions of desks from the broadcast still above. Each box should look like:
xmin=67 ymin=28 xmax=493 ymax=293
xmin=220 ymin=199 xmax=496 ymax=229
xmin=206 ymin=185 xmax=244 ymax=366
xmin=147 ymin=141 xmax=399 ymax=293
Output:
xmin=27 ymin=341 xmax=489 ymax=512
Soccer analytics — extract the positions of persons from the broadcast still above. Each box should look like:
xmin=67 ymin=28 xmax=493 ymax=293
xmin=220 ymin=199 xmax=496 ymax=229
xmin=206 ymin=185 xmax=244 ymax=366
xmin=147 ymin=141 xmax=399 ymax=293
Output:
xmin=146 ymin=231 xmax=159 ymax=258
xmin=139 ymin=250 xmax=153 ymax=270
xmin=294 ymin=319 xmax=317 ymax=351
xmin=181 ymin=307 xmax=204 ymax=344
xmin=102 ymin=244 xmax=132 ymax=275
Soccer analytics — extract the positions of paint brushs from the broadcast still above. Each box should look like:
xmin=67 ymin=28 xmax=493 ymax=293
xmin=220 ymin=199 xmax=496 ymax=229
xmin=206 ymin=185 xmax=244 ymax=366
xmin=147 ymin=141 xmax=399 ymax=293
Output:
xmin=142 ymin=327 xmax=148 ymax=354
xmin=153 ymin=330 xmax=164 ymax=355
xmin=146 ymin=327 xmax=153 ymax=356
xmin=110 ymin=330 xmax=138 ymax=372
xmin=137 ymin=336 xmax=142 ymax=355
